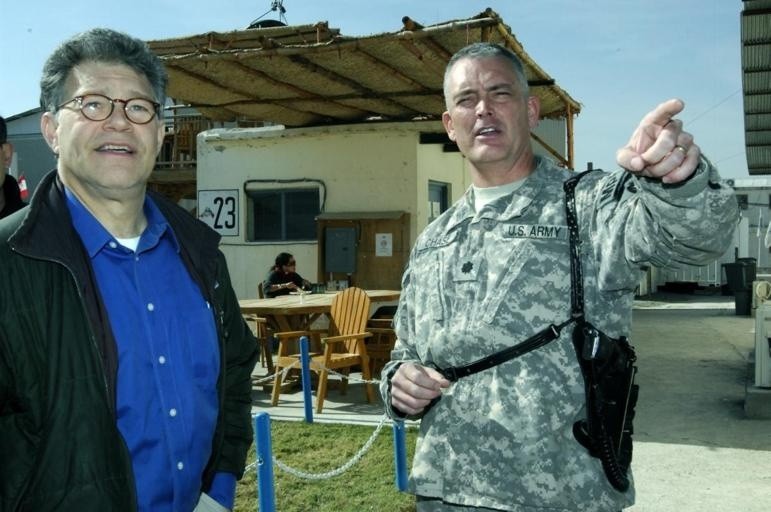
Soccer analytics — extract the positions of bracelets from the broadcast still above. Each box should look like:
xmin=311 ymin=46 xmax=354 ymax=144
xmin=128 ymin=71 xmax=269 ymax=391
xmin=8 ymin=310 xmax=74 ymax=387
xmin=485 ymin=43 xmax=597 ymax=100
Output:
xmin=278 ymin=283 xmax=285 ymax=290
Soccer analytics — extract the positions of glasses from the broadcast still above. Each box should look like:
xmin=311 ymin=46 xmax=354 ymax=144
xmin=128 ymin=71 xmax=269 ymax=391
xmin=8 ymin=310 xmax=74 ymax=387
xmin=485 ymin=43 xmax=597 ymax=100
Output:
xmin=55 ymin=92 xmax=160 ymax=124
xmin=285 ymin=260 xmax=295 ymax=267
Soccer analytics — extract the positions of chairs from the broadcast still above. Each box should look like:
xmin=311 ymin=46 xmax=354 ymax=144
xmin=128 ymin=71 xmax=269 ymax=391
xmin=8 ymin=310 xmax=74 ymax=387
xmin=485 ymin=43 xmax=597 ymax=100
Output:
xmin=257 ymin=277 xmax=314 ymax=358
xmin=268 ymin=286 xmax=378 ymax=412
xmin=363 ymin=324 xmax=397 ymax=373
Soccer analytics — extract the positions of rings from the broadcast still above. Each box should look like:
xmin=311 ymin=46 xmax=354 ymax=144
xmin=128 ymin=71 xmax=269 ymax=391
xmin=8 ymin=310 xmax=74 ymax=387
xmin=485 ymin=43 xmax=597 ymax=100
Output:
xmin=675 ymin=144 xmax=687 ymax=156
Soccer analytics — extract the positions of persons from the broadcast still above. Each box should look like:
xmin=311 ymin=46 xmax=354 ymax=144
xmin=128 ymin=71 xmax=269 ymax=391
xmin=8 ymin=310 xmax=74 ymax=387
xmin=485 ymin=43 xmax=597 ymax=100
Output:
xmin=381 ymin=44 xmax=742 ymax=512
xmin=0 ymin=114 xmax=32 ymax=216
xmin=264 ymin=253 xmax=312 ymax=348
xmin=0 ymin=29 xmax=262 ymax=512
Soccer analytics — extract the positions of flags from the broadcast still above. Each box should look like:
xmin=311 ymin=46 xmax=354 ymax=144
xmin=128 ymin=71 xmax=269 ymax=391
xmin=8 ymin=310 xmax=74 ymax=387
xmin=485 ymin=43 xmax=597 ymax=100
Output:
xmin=18 ymin=172 xmax=30 ymax=200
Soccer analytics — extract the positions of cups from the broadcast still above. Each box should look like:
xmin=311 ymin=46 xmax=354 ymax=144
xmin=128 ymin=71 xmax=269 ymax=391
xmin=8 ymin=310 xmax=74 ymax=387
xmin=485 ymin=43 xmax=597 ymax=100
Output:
xmin=327 ymin=280 xmax=349 ymax=291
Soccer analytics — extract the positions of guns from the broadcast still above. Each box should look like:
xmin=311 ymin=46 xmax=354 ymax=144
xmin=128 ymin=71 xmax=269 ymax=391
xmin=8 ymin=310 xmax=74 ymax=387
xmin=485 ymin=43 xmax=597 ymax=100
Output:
xmin=580 ymin=327 xmax=639 ymax=472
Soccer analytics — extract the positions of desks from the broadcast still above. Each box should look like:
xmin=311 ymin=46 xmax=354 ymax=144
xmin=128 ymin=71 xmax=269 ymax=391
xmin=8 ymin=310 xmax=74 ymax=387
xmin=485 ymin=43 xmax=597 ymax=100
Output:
xmin=237 ymin=289 xmax=399 ymax=392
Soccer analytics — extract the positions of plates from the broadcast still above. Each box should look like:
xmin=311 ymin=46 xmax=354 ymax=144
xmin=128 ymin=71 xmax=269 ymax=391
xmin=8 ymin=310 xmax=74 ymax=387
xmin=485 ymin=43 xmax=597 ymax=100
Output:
xmin=288 ymin=291 xmax=312 ymax=295
